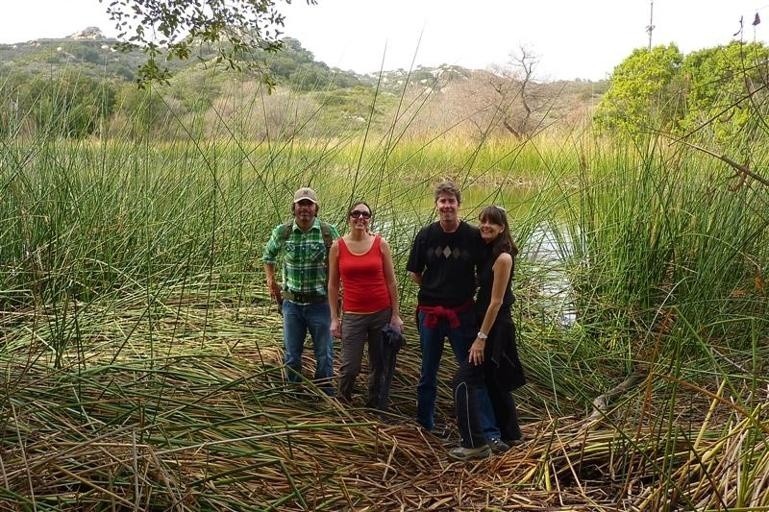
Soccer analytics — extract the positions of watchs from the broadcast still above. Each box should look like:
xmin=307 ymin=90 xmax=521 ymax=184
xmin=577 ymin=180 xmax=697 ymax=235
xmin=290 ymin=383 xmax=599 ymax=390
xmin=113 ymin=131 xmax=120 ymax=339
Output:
xmin=476 ymin=330 xmax=487 ymax=339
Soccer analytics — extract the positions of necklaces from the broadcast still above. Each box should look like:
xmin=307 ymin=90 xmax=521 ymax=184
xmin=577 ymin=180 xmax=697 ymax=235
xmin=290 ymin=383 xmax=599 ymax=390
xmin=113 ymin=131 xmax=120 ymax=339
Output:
xmin=346 ymin=231 xmax=370 ymax=251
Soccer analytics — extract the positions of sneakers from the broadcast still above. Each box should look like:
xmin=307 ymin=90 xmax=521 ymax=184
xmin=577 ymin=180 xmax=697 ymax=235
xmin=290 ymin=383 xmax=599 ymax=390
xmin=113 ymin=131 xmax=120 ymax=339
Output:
xmin=447 ymin=444 xmax=491 ymax=460
xmin=489 ymin=437 xmax=509 ymax=454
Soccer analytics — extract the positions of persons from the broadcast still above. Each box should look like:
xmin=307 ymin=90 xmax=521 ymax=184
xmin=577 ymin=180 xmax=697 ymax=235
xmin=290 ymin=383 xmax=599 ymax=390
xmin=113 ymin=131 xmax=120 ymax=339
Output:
xmin=444 ymin=205 xmax=527 ymax=462
xmin=406 ymin=181 xmax=483 ymax=432
xmin=328 ymin=201 xmax=402 ymax=406
xmin=263 ymin=187 xmax=342 ymax=395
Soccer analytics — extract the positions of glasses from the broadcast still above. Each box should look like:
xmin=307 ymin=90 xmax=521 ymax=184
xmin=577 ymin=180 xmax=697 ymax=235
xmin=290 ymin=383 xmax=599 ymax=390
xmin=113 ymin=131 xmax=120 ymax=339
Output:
xmin=349 ymin=210 xmax=370 ymax=220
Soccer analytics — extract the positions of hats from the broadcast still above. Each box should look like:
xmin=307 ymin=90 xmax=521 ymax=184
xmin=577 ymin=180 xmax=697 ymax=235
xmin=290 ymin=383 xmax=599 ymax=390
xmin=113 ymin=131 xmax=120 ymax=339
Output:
xmin=293 ymin=187 xmax=317 ymax=204
